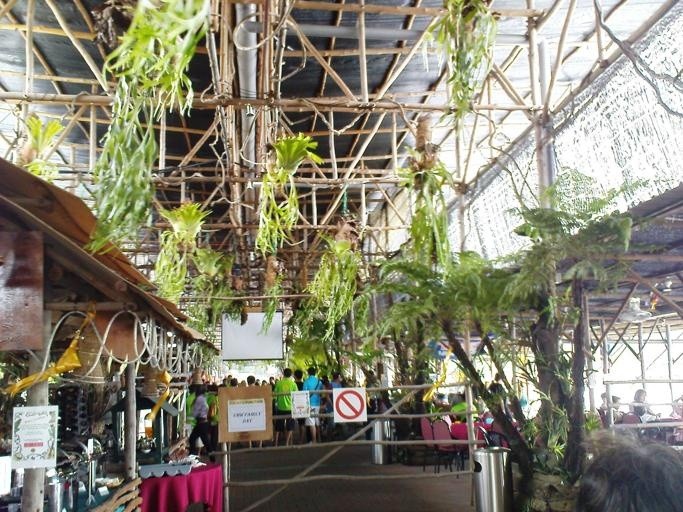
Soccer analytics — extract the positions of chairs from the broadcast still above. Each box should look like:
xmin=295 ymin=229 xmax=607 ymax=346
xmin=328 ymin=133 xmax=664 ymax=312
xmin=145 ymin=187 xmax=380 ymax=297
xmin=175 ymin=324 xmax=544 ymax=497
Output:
xmin=597 ymin=408 xmax=642 ymax=431
xmin=419 ymin=416 xmax=511 ymax=478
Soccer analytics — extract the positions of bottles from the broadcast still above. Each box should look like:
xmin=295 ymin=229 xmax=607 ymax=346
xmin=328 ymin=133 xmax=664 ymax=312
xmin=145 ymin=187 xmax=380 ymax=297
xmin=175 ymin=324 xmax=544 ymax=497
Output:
xmin=47 ymin=473 xmax=79 ymax=512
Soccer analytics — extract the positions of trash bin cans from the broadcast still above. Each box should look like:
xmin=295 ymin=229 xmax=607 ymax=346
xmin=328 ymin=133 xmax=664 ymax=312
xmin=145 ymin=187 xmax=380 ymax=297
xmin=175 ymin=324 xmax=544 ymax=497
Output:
xmin=370 ymin=419 xmax=398 ymax=465
xmin=471 ymin=446 xmax=515 ymax=512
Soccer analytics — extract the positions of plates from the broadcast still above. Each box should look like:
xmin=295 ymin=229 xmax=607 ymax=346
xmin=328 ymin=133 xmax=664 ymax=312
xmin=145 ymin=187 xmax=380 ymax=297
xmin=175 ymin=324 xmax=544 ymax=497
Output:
xmin=95 ymin=477 xmax=125 ymax=487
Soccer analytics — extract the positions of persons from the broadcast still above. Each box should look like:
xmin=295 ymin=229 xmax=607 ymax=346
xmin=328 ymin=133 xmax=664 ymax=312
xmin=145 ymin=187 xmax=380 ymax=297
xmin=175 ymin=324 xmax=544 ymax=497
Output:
xmin=572 ymin=430 xmax=682 ymax=512
xmin=188 ymin=367 xmax=379 ymax=455
xmin=450 ymin=373 xmax=511 ymax=421
xmin=600 ymin=388 xmax=682 ymax=422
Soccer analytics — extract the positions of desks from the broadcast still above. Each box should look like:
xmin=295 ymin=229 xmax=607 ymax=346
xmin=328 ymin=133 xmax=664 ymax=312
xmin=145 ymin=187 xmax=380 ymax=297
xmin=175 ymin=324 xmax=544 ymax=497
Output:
xmin=138 ymin=462 xmax=223 ymax=512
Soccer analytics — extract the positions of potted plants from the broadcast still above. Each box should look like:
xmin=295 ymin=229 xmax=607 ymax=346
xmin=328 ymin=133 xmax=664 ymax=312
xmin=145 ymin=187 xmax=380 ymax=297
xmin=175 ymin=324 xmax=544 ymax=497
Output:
xmin=377 ymin=201 xmax=638 ymax=512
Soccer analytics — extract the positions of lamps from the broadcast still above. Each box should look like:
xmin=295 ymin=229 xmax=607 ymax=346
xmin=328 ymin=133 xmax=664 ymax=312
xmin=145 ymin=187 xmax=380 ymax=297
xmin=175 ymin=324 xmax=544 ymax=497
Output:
xmin=69 ymin=320 xmax=106 ymax=384
